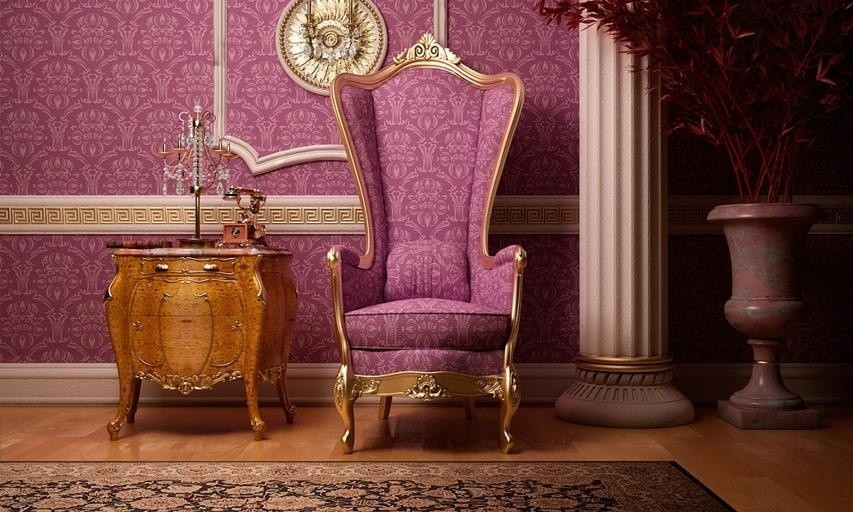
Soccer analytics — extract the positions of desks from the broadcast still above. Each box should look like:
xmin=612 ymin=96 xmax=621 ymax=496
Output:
xmin=103 ymin=246 xmax=300 ymax=443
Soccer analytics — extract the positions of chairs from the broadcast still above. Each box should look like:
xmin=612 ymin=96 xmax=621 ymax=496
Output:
xmin=326 ymin=28 xmax=530 ymax=455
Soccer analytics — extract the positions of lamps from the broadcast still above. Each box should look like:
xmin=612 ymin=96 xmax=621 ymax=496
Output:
xmin=156 ymin=106 xmax=234 ymax=245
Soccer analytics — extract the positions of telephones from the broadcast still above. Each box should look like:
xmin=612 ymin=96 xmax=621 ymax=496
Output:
xmin=222 ymin=185 xmax=268 ymax=249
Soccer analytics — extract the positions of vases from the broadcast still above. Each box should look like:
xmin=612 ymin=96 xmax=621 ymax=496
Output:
xmin=706 ymin=201 xmax=823 ymax=431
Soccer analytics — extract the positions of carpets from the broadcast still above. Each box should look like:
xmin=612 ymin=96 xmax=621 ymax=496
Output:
xmin=2 ymin=460 xmax=744 ymax=511
